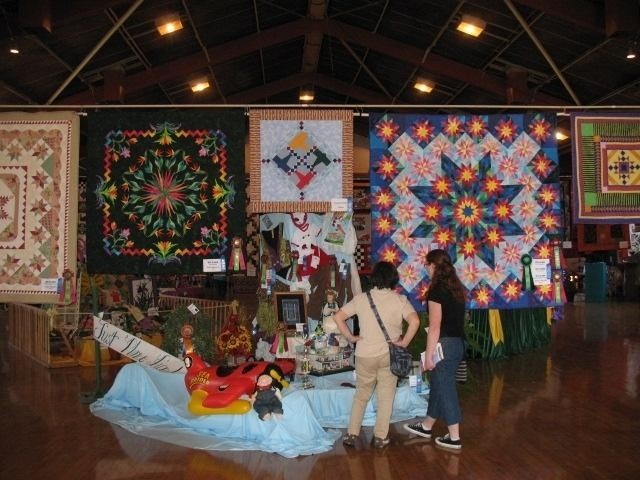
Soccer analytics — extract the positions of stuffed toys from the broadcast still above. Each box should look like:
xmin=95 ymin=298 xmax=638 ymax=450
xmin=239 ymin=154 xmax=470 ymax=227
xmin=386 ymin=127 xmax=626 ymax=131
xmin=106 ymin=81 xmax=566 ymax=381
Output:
xmin=181 ymin=351 xmax=291 ymax=415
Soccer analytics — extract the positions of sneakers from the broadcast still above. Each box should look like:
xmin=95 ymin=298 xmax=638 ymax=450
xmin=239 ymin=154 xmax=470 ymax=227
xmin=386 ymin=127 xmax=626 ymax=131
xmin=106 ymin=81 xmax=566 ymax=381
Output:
xmin=435 ymin=432 xmax=462 ymax=449
xmin=374 ymin=437 xmax=390 ymax=448
xmin=403 ymin=422 xmax=432 ymax=439
xmin=343 ymin=433 xmax=357 ymax=449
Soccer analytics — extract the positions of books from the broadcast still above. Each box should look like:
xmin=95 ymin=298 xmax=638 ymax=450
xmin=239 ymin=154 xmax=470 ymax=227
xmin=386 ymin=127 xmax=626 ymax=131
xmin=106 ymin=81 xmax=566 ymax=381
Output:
xmin=418 ymin=342 xmax=444 ymax=372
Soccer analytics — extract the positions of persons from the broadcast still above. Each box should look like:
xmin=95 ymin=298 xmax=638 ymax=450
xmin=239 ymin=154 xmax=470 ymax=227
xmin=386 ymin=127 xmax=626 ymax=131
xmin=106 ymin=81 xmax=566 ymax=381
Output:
xmin=402 ymin=249 xmax=467 ymax=450
xmin=333 ymin=262 xmax=421 ymax=450
xmin=318 ymin=286 xmax=346 ymax=333
xmin=254 ymin=372 xmax=284 ymax=423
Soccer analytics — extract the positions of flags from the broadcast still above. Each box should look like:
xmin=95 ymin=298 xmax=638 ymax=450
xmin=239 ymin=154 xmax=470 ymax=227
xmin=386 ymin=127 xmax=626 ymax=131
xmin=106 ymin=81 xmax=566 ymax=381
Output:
xmin=81 ymin=108 xmax=248 ymax=277
xmin=245 ymin=108 xmax=355 ymax=213
xmin=568 ymin=111 xmax=640 ymax=225
xmin=365 ymin=113 xmax=569 ymax=310
xmin=1 ymin=110 xmax=82 ymax=307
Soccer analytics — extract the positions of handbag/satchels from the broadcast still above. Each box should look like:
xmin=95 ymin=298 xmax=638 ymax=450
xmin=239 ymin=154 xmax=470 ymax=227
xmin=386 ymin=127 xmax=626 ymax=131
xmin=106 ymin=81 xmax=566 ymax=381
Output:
xmin=455 ymin=360 xmax=468 ymax=384
xmin=390 ymin=344 xmax=413 ymax=378
